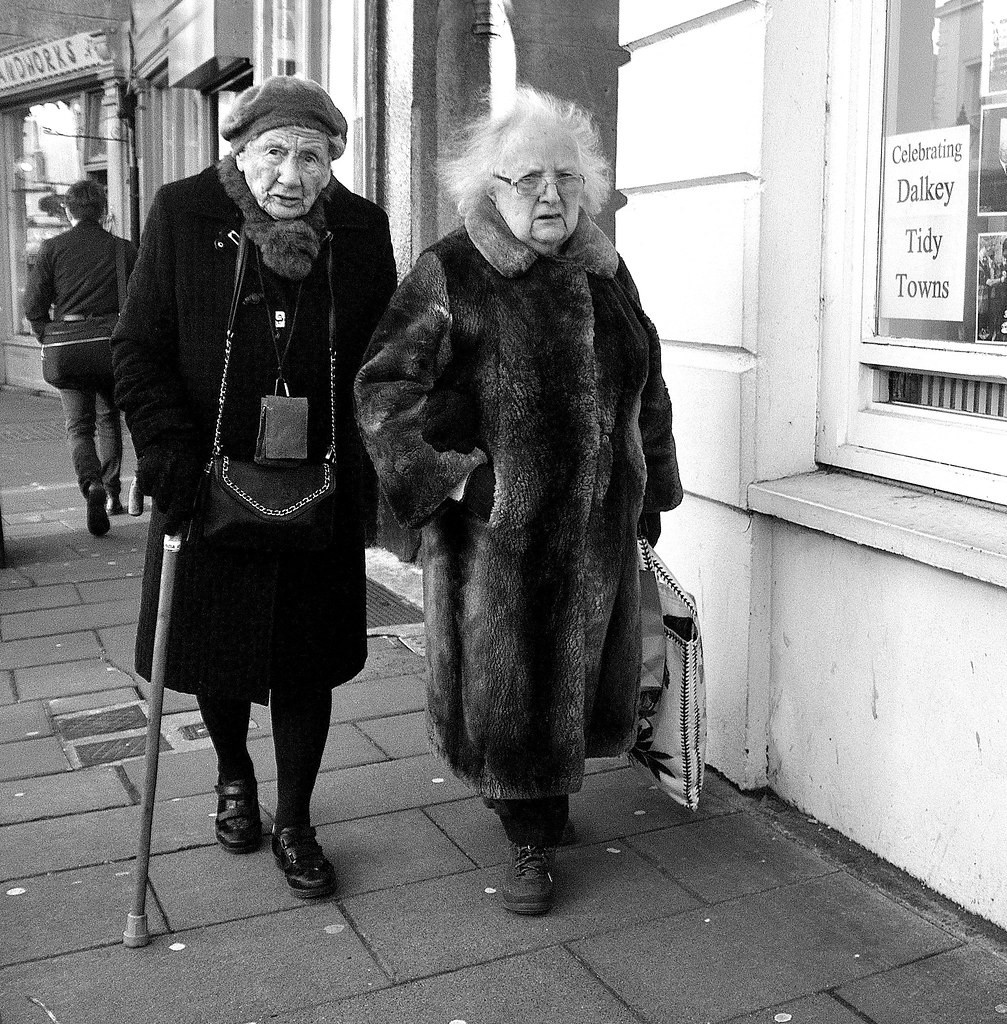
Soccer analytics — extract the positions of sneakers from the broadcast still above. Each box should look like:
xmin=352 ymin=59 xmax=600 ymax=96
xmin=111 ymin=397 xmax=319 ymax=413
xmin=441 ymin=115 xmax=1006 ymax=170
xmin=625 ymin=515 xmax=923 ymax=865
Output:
xmin=501 ymin=839 xmax=557 ymax=917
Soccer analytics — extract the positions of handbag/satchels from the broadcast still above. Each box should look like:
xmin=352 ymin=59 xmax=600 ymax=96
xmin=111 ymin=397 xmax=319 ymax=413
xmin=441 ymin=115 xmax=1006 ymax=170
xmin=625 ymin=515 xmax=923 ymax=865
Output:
xmin=38 ymin=314 xmax=119 ymax=390
xmin=202 ymin=457 xmax=339 ymax=539
xmin=632 ymin=543 xmax=708 ymax=814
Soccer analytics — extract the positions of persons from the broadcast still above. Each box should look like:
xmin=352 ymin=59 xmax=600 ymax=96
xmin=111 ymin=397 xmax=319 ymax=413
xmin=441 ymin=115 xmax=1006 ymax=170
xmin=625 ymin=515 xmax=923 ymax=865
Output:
xmin=107 ymin=76 xmax=399 ymax=897
xmin=23 ymin=179 xmax=140 ymax=535
xmin=354 ymin=78 xmax=686 ymax=917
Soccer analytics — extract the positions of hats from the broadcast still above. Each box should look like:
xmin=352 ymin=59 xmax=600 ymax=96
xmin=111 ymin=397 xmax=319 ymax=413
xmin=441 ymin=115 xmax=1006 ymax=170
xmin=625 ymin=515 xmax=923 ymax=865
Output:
xmin=220 ymin=76 xmax=349 ymax=161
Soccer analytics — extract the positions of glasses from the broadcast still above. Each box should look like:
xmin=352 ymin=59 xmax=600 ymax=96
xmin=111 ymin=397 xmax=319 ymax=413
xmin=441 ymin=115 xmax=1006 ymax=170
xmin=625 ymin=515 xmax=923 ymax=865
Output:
xmin=491 ymin=172 xmax=592 ymax=200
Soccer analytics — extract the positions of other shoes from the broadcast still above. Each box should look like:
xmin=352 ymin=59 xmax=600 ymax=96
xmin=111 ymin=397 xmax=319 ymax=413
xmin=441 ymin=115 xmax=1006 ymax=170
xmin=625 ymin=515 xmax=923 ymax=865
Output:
xmin=86 ymin=484 xmax=110 ymax=536
xmin=106 ymin=497 xmax=123 ymax=514
xmin=212 ymin=774 xmax=263 ymax=854
xmin=269 ymin=823 xmax=338 ymax=901
